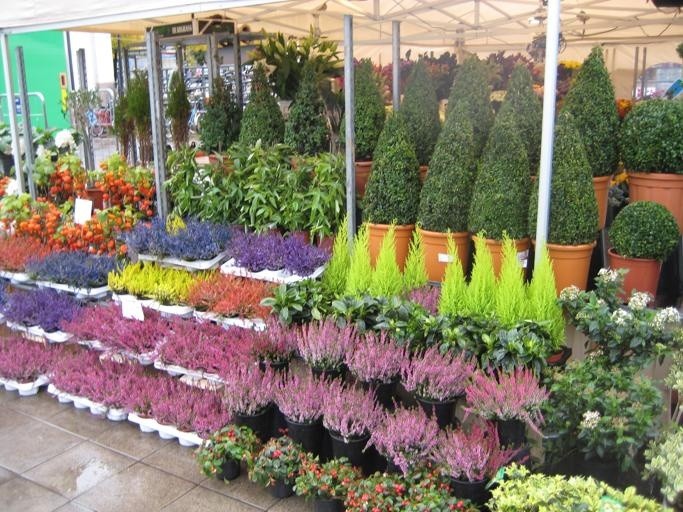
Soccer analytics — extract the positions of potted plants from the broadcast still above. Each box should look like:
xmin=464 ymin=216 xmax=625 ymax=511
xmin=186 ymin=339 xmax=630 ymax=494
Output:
xmin=335 ymin=56 xmax=388 ymax=200
xmin=616 ymin=99 xmax=682 ymax=235
xmin=396 ymin=57 xmax=442 ymax=187
xmin=526 ymin=243 xmax=569 ymax=367
xmin=412 ymin=101 xmax=480 ymax=282
xmin=478 ymin=60 xmax=537 ymax=187
xmin=59 ymin=86 xmax=105 ymax=212
xmin=526 ymin=111 xmax=600 ymax=300
xmin=361 ymin=111 xmax=423 ymax=273
xmin=465 ymin=97 xmax=537 ymax=284
xmin=608 ymin=201 xmax=680 ymax=307
xmin=248 ymin=26 xmax=344 ymax=121
xmin=561 ymin=44 xmax=619 ymax=230
xmin=195 ymin=103 xmax=228 ymax=158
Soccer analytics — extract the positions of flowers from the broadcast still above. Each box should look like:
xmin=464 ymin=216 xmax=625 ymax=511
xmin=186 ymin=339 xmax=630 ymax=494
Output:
xmin=192 ymin=422 xmax=258 ymax=479
xmin=529 ymin=266 xmax=680 ymax=473
xmin=460 ymin=358 xmax=552 ymax=437
xmin=155 ymin=314 xmax=269 ymax=382
xmin=60 ymin=294 xmax=163 ymax=353
xmin=640 ymin=346 xmax=683 ymax=511
xmin=246 ymin=429 xmax=314 ymax=489
xmin=361 ymin=395 xmax=440 ymax=477
xmin=294 ymin=319 xmax=355 ymax=372
xmin=0 ymin=285 xmax=80 ymax=333
xmin=0 ymin=236 xmax=51 ymax=273
xmin=31 ymin=247 xmax=116 ymax=290
xmin=0 ymin=325 xmax=229 ymax=435
xmin=322 ymin=377 xmax=386 ymax=444
xmin=291 ymin=456 xmax=363 ymax=503
xmin=187 ymin=271 xmax=272 ymax=321
xmin=227 ymin=222 xmax=328 ymax=281
xmin=106 ymin=260 xmax=215 ymax=310
xmin=400 ymin=340 xmax=478 ymax=402
xmin=120 ymin=214 xmax=230 ymax=260
xmin=427 ymin=423 xmax=529 ymax=483
xmin=343 ymin=329 xmax=404 ymax=383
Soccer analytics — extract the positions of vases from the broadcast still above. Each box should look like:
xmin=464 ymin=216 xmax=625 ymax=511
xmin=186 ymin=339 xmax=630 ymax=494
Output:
xmin=34 ymin=280 xmax=111 ymax=301
xmin=387 ymin=457 xmax=404 ymax=475
xmin=258 ymin=358 xmax=289 ymax=375
xmin=361 ymin=380 xmax=397 ymax=406
xmin=4 ymin=320 xmax=75 ymax=347
xmin=265 ymin=478 xmax=299 ymax=499
xmin=112 ymin=293 xmax=192 ymax=317
xmin=574 ymin=435 xmax=662 ymax=503
xmin=76 ymin=329 xmax=175 ymax=366
xmin=129 ymin=247 xmax=227 ymax=274
xmin=310 ymin=365 xmax=344 ymax=378
xmin=218 ymin=461 xmax=241 ymax=481
xmin=234 ymin=408 xmax=272 ymax=444
xmin=128 ymin=411 xmax=212 ymax=447
xmin=0 ymin=268 xmax=29 ymax=287
xmin=317 ymin=498 xmax=343 ymax=512
xmin=194 ymin=308 xmax=267 ymax=332
xmin=487 ymin=420 xmax=525 ymax=447
xmin=0 ymin=370 xmax=54 ymax=396
xmin=218 ymin=257 xmax=328 ymax=285
xmin=283 ymin=417 xmax=321 ymax=458
xmin=151 ymin=356 xmax=231 ymax=386
xmin=415 ymin=394 xmax=458 ymax=430
xmin=446 ymin=476 xmax=488 ymax=504
xmin=46 ymin=384 xmax=129 ymax=423
xmin=329 ymin=428 xmax=370 ymax=468
xmin=86 ymin=190 xmax=104 ymax=216
